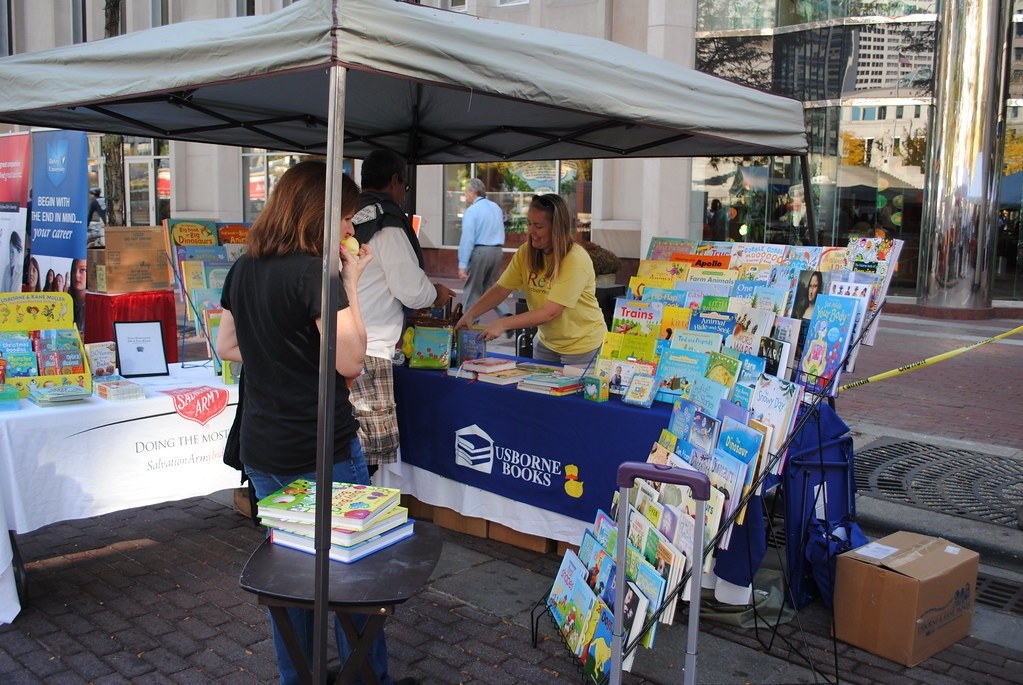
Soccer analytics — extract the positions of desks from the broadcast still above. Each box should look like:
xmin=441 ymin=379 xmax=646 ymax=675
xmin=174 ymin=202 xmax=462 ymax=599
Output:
xmin=371 ymin=352 xmax=852 ymax=606
xmin=238 ymin=517 xmax=442 ymax=685
xmin=0 ymin=358 xmax=250 ymax=627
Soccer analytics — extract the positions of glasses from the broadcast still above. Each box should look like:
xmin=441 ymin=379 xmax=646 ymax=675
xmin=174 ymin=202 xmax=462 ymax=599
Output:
xmin=401 ymin=180 xmax=410 ymax=192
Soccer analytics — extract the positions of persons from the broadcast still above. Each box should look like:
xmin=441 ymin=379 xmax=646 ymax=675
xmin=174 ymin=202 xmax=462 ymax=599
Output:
xmin=454 ymin=194 xmax=608 ymax=363
xmin=708 ymin=199 xmax=726 ymax=241
xmin=338 ymin=152 xmax=456 ymax=465
xmin=217 ymin=160 xmax=415 ymax=685
xmin=999 ymin=211 xmax=1003 ymax=229
xmin=26 ymin=189 xmax=32 ymax=248
xmin=458 ymin=179 xmax=514 ymax=338
xmin=88 ymin=188 xmax=106 ymax=228
xmin=29 ymin=256 xmax=87 ymax=331
xmin=609 ymin=366 xmax=622 ymax=389
xmin=2 ymin=231 xmax=22 ymax=291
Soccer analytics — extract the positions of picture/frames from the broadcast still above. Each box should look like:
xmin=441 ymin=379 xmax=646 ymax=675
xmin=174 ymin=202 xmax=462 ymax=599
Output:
xmin=113 ymin=319 xmax=170 ymax=378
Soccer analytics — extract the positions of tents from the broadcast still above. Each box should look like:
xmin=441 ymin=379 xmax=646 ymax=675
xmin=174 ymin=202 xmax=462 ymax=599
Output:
xmin=1000 ymin=170 xmax=1023 ymax=210
xmin=0 ymin=0 xmax=819 ymax=685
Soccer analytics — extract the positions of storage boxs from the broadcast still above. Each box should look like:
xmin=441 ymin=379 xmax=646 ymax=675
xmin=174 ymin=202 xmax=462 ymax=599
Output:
xmin=86 ymin=226 xmax=170 ymax=295
xmin=488 ymin=521 xmax=557 ymax=554
xmin=557 ymin=541 xmax=580 ymax=557
xmin=401 ymin=494 xmax=434 ymax=519
xmin=0 ymin=401 xmax=20 ymax=412
xmin=433 ymin=506 xmax=489 ymax=538
xmin=831 ymin=530 xmax=980 ymax=668
xmin=584 ymin=375 xmax=610 ymax=403
xmin=0 ymin=291 xmax=92 ymax=398
xmin=0 ymin=384 xmax=20 ymax=401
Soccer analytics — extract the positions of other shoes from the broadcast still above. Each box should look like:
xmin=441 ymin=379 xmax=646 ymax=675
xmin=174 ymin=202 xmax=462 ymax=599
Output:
xmin=505 ymin=313 xmax=514 ymax=339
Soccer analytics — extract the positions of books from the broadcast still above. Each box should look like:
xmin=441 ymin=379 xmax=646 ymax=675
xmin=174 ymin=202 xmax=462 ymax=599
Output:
xmin=84 ymin=341 xmax=145 ymax=400
xmin=163 ymin=220 xmax=255 ymax=385
xmin=547 ymin=238 xmax=904 ymax=685
xmin=409 ymin=326 xmax=453 ymax=369
xmin=0 ymin=329 xmax=84 ymax=378
xmin=0 ymin=384 xmax=92 ymax=410
xmin=257 ymin=478 xmax=415 ymax=563
xmin=447 ymin=325 xmax=584 ymax=396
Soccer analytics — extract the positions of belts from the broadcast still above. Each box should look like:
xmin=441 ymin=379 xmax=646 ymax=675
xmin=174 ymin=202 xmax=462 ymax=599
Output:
xmin=475 ymin=244 xmax=502 ymax=247
